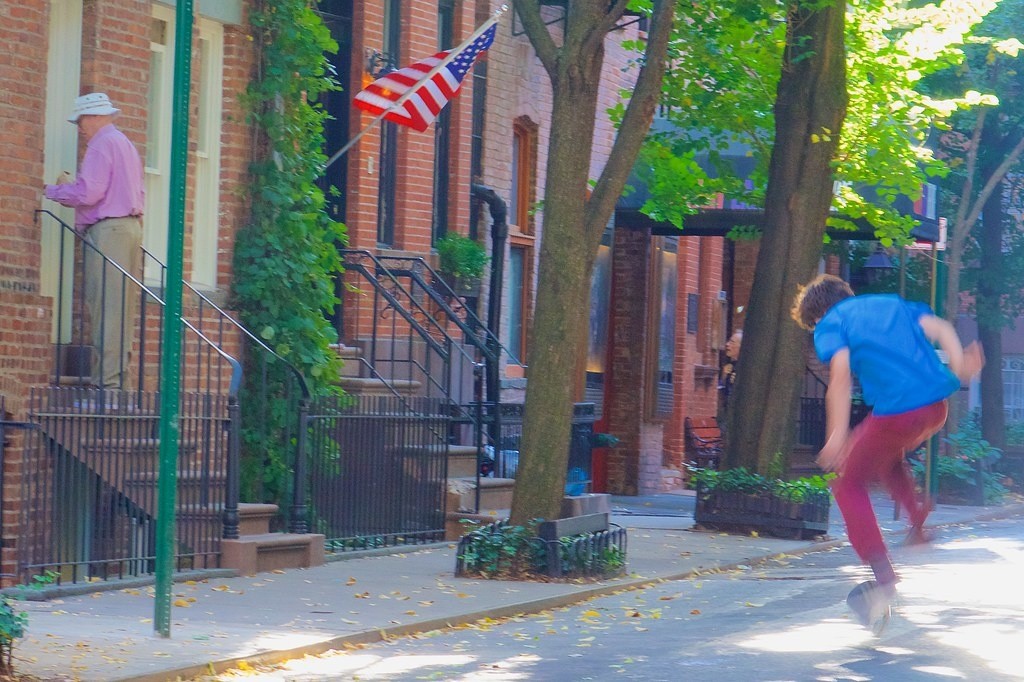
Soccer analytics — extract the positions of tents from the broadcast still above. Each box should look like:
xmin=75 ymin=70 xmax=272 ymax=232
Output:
xmin=651 ymin=207 xmax=942 ymax=521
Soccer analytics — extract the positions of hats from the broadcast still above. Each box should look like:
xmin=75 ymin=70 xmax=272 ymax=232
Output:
xmin=67 ymin=92 xmax=120 ymax=124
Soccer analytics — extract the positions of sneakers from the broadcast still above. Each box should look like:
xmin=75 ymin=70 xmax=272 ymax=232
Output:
xmin=73 ymin=394 xmax=138 ymax=415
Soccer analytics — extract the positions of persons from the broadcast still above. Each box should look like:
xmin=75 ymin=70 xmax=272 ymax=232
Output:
xmin=717 ymin=329 xmax=743 ymax=412
xmin=791 ymin=272 xmax=964 ymax=605
xmin=42 ymin=92 xmax=146 ymax=389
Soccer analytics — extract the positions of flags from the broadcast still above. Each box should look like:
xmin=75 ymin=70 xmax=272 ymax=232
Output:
xmin=353 ymin=22 xmax=498 ymax=130
